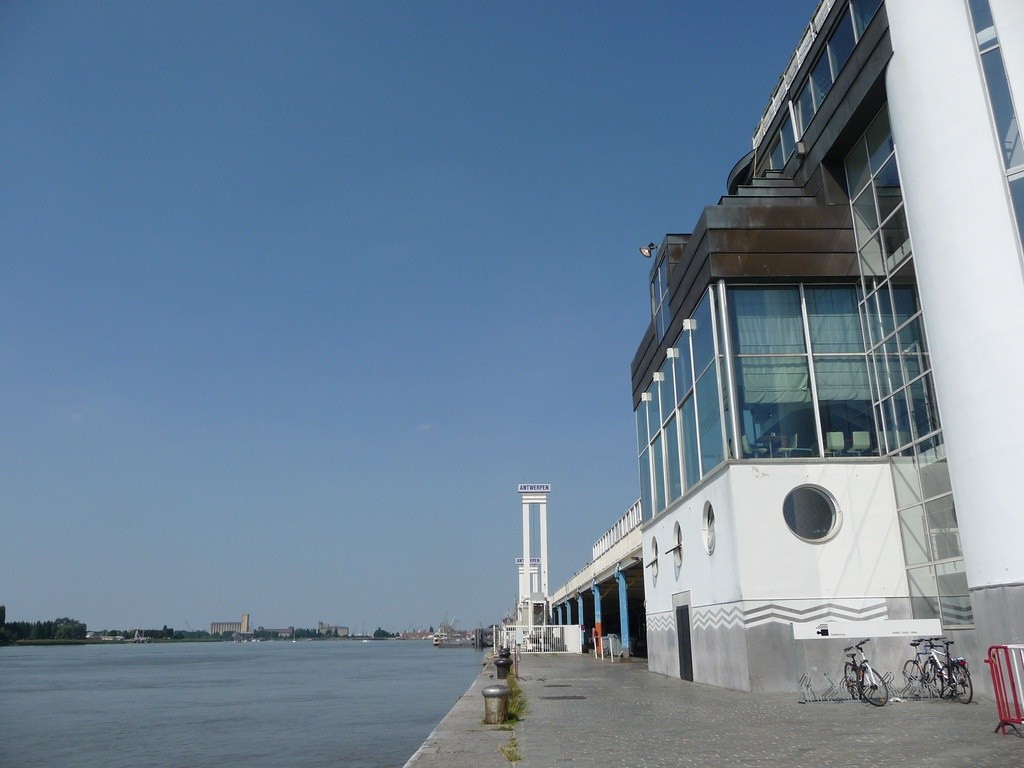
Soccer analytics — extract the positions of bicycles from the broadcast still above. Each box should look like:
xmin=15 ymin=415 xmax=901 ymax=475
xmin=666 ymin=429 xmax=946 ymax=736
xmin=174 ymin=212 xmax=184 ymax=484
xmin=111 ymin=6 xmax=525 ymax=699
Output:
xmin=903 ymin=636 xmax=974 ymax=705
xmin=844 ymin=638 xmax=888 ymax=707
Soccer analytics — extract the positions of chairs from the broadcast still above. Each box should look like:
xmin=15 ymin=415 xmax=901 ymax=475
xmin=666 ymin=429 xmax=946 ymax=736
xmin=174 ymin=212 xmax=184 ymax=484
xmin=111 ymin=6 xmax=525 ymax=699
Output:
xmin=873 ymin=430 xmax=911 ymax=458
xmin=742 ymin=434 xmax=767 ymax=458
xmin=848 ymin=431 xmax=871 ymax=457
xmin=824 ymin=431 xmax=845 ymax=457
xmin=776 ymin=432 xmax=798 ymax=457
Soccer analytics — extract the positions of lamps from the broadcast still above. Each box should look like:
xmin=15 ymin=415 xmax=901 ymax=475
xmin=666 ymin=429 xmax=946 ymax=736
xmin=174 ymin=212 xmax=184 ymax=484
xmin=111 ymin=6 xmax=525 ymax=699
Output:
xmin=639 ymin=241 xmax=660 ymax=258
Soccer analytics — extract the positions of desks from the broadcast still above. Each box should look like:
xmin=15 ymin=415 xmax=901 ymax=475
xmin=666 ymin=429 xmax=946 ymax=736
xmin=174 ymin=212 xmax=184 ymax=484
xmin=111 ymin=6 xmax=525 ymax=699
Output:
xmin=757 ymin=434 xmax=788 ymax=457
